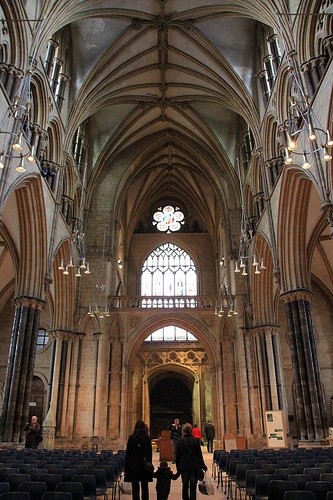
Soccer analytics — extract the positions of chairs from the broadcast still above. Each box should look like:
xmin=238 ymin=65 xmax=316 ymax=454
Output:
xmin=0 ymin=448 xmax=126 ymax=500
xmin=212 ymin=447 xmax=333 ymax=500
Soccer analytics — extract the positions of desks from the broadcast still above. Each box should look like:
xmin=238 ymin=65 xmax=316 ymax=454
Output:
xmin=224 ymin=431 xmax=247 ymax=453
xmin=159 ymin=437 xmax=173 ymax=462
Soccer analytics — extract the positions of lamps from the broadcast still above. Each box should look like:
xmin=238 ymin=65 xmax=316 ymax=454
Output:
xmin=0 ymin=18 xmax=44 ymax=173
xmin=87 ymin=282 xmax=110 ymax=318
xmin=275 ymin=12 xmax=333 ymax=169
xmin=214 ymin=274 xmax=238 ymax=317
xmin=233 ymin=157 xmax=266 ymax=276
xmin=57 ymin=164 xmax=91 ymax=277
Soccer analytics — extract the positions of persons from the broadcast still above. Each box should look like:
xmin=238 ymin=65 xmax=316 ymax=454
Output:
xmin=152 ymin=462 xmax=180 ymax=500
xmin=124 ymin=421 xmax=152 ymax=500
xmin=205 ymin=419 xmax=215 ymax=453
xmin=171 ymin=419 xmax=182 ymax=464
xmin=191 ymin=424 xmax=202 ymax=445
xmin=175 ymin=423 xmax=208 ymax=500
xmin=23 ymin=416 xmax=40 ymax=449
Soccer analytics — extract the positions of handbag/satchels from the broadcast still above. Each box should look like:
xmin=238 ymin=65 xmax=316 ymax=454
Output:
xmin=145 ymin=462 xmax=154 ymax=473
xmin=199 ymin=470 xmax=216 ymax=495
